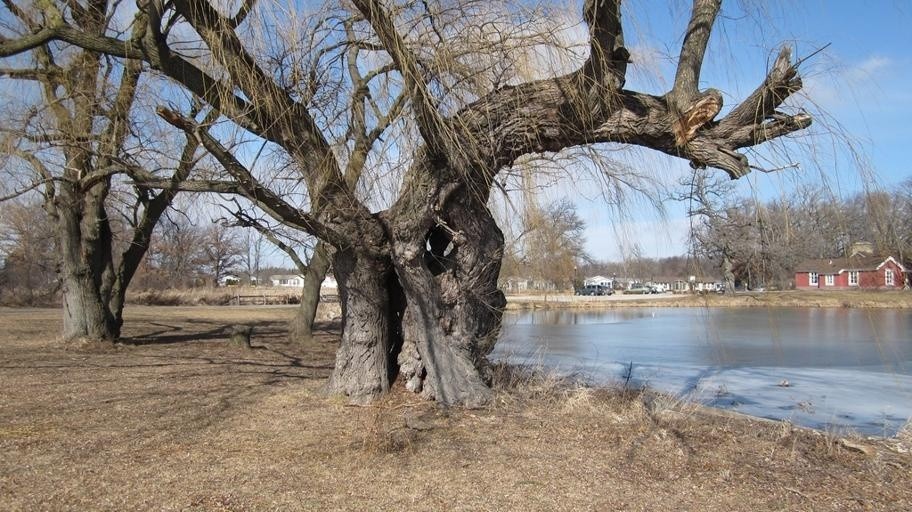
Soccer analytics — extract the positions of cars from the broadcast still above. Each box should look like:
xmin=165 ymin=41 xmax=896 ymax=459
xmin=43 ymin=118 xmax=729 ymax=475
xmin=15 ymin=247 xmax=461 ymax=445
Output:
xmin=623 ymin=285 xmax=666 ymax=294
xmin=574 ymin=285 xmax=615 ymax=296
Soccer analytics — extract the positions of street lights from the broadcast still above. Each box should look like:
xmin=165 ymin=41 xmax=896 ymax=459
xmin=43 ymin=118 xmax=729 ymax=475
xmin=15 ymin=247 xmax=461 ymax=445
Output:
xmin=574 ymin=264 xmax=577 ymax=284
xmin=613 ymin=272 xmax=616 ymax=290
